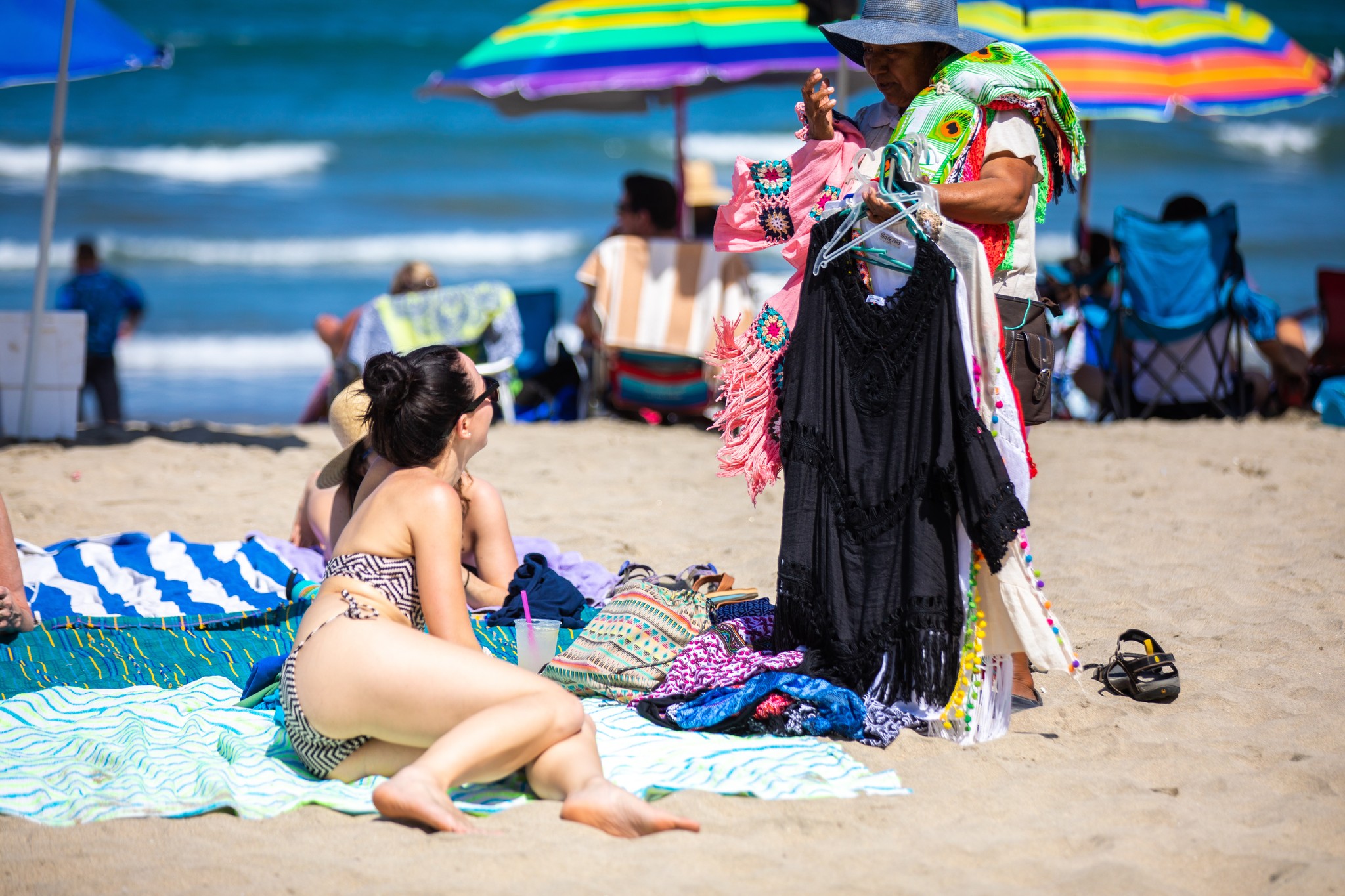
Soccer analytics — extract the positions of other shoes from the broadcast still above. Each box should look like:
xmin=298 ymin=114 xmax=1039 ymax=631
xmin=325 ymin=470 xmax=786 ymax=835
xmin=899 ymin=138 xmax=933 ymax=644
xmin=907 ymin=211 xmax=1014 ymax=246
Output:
xmin=1008 ymin=687 xmax=1043 ymax=719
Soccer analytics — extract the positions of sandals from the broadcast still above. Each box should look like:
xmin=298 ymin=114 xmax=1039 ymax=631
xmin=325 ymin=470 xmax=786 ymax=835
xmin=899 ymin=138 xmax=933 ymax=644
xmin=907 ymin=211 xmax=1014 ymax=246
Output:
xmin=619 ymin=562 xmax=659 ymax=586
xmin=673 ymin=562 xmax=731 ymax=596
xmin=1091 ymin=629 xmax=1181 ymax=701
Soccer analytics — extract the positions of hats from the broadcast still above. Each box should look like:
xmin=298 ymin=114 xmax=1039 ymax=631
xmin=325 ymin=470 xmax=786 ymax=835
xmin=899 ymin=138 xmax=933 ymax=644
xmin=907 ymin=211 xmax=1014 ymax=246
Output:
xmin=315 ymin=379 xmax=374 ymax=489
xmin=399 ymin=259 xmax=437 ymax=289
xmin=818 ymin=0 xmax=998 ymax=69
xmin=681 ymin=158 xmax=733 ymax=208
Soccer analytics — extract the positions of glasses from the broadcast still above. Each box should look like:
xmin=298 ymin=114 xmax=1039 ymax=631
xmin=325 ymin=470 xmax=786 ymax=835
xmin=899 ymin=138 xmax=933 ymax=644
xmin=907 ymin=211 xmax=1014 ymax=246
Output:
xmin=441 ymin=375 xmax=500 ymax=440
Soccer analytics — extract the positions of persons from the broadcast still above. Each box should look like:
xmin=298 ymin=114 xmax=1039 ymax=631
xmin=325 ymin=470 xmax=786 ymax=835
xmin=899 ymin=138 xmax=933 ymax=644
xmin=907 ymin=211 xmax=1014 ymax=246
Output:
xmin=1107 ymin=194 xmax=1274 ymax=416
xmin=58 ymin=235 xmax=147 ymax=442
xmin=791 ymin=0 xmax=1060 ymax=714
xmin=298 ymin=259 xmax=439 ymax=419
xmin=0 ymin=482 xmax=35 ymax=639
xmin=280 ymin=344 xmax=703 ymax=836
xmin=576 ymin=169 xmax=697 ymax=424
xmin=292 ymin=377 xmax=522 ymax=612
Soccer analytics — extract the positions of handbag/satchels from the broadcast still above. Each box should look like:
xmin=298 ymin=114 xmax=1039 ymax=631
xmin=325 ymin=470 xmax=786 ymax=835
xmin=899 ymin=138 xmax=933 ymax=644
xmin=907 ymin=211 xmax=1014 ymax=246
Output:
xmin=537 ymin=574 xmax=758 ymax=704
xmin=994 ymin=292 xmax=1062 ymax=426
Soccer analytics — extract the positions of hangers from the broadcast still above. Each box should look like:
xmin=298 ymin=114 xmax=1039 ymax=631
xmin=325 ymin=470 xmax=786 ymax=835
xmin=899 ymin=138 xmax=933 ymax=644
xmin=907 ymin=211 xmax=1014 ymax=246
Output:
xmin=812 ymin=134 xmax=988 ymax=282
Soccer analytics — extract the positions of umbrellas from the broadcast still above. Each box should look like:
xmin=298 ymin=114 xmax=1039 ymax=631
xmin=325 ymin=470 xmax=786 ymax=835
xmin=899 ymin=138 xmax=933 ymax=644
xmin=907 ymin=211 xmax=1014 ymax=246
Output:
xmin=944 ymin=0 xmax=1321 ymax=299
xmin=1 ymin=2 xmax=178 ymax=87
xmin=414 ymin=0 xmax=869 ymax=237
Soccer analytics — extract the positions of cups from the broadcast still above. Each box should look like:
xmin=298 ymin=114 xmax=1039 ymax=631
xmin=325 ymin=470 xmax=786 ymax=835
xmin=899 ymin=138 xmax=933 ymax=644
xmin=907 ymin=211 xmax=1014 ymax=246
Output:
xmin=514 ymin=618 xmax=563 ymax=673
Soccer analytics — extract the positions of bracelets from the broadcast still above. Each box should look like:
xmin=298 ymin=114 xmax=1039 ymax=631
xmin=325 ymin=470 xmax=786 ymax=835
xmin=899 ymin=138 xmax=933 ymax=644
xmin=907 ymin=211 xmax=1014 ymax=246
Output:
xmin=464 ymin=568 xmax=471 ymax=588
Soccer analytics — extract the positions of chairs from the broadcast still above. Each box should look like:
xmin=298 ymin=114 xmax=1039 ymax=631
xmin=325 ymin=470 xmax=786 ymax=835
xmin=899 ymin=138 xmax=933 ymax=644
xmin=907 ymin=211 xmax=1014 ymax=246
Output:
xmin=549 ymin=234 xmax=750 ymax=416
xmin=1078 ymin=203 xmax=1276 ymax=424
xmin=332 ymin=280 xmax=525 ymax=426
